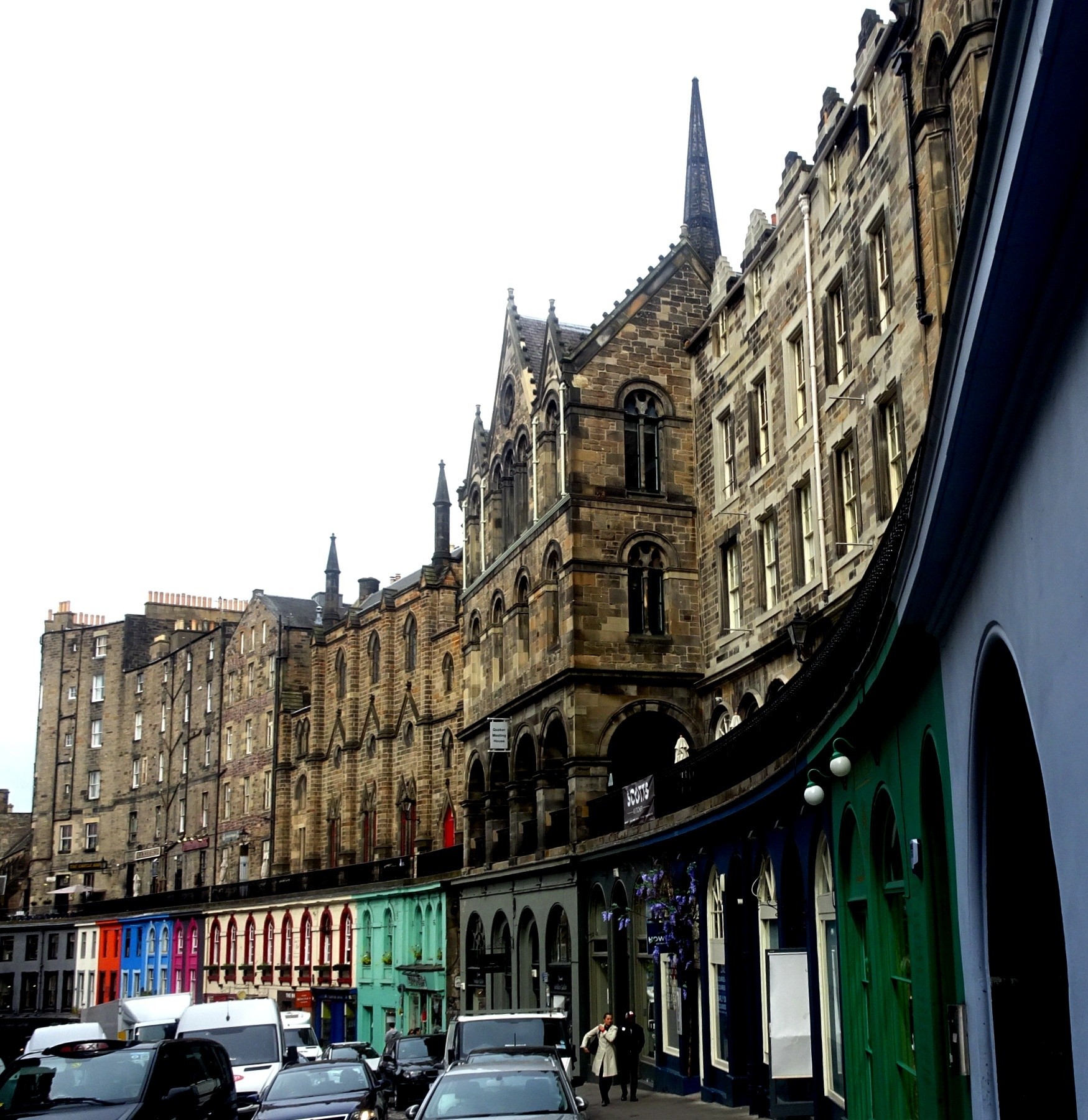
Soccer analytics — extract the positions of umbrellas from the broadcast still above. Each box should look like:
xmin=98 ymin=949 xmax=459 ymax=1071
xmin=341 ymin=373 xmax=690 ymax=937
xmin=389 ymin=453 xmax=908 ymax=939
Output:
xmin=46 ymin=884 xmax=106 ymax=905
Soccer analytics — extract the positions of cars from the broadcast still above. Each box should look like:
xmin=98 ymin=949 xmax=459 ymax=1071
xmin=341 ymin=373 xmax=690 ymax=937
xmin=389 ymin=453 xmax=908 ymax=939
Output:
xmin=0 ymin=993 xmax=586 ymax=1120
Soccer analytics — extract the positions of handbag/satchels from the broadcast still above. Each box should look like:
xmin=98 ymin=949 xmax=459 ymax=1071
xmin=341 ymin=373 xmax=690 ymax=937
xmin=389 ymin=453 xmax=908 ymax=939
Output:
xmin=587 ymin=1024 xmax=603 ymax=1055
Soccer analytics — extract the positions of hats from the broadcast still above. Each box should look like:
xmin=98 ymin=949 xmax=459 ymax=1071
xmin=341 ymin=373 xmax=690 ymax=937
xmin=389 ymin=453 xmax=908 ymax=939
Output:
xmin=624 ymin=1011 xmax=636 ymax=1019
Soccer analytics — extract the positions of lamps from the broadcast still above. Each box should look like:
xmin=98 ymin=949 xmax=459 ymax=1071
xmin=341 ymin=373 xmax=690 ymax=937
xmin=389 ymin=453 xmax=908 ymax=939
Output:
xmin=786 ymin=605 xmax=809 ymax=664
xmin=401 ymin=793 xmax=420 ymax=822
xmin=239 ymin=826 xmax=255 ymax=850
xmin=804 ymin=769 xmax=829 ymax=805
xmin=99 ymin=856 xmax=112 ymax=876
xmin=829 ymin=737 xmax=856 ymax=777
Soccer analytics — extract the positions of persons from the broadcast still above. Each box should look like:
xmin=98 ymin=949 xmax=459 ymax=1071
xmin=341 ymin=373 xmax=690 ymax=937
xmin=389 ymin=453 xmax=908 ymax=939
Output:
xmin=580 ymin=1012 xmax=619 ymax=1107
xmin=432 ymin=1018 xmax=442 ymax=1033
xmin=616 ymin=1011 xmax=646 ymax=1102
xmin=384 ymin=1022 xmax=400 ymax=1044
xmin=413 ymin=1027 xmax=421 ymax=1035
xmin=407 ymin=1028 xmax=414 ymax=1036
xmin=68 ymin=893 xmax=86 ymax=917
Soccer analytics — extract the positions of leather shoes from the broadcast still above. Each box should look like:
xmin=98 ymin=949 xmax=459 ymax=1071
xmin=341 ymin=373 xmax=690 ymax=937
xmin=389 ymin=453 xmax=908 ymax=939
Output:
xmin=630 ymin=1096 xmax=638 ymax=1102
xmin=621 ymin=1092 xmax=627 ymax=1101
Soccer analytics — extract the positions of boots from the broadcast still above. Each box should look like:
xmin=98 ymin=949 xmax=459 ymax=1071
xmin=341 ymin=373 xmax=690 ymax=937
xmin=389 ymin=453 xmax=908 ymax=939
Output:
xmin=600 ymin=1093 xmax=606 ymax=1106
xmin=605 ymin=1093 xmax=610 ymax=1103
